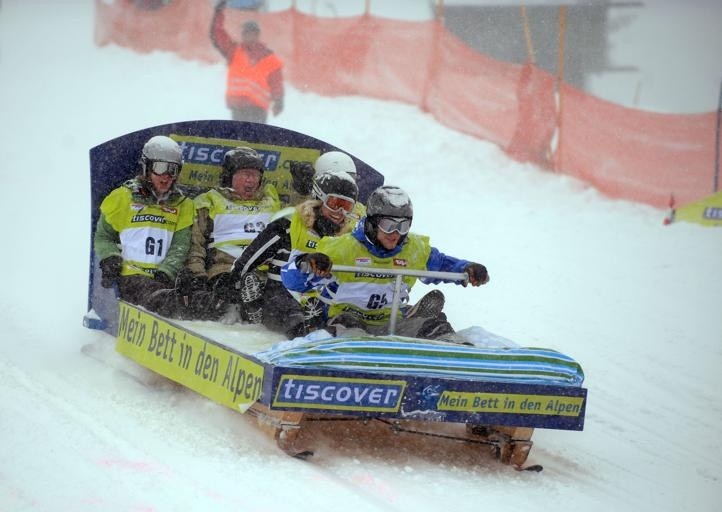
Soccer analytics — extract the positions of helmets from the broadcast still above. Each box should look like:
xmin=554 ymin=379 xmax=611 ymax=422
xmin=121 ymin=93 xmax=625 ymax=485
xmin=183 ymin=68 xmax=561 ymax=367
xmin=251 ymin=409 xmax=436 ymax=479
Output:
xmin=137 ymin=135 xmax=185 ymax=178
xmin=366 ymin=186 xmax=413 ymax=227
xmin=219 ymin=145 xmax=265 ymax=189
xmin=311 ymin=151 xmax=359 ymax=205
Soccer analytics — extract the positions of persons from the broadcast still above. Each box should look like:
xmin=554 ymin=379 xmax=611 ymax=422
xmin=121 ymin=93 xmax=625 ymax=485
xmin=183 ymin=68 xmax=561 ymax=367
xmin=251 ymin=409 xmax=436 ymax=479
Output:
xmin=279 ymin=185 xmax=488 ymax=347
xmin=93 ymin=136 xmax=233 ymax=322
xmin=208 ymin=0 xmax=283 ymax=125
xmin=288 ymin=150 xmax=367 ymax=219
xmin=186 ymin=146 xmax=316 ymax=341
xmin=214 ymin=170 xmax=444 ymax=340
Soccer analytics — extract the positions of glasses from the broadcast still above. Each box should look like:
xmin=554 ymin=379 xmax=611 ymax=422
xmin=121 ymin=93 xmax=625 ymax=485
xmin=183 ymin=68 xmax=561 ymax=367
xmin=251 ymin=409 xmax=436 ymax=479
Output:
xmin=137 ymin=153 xmax=180 ymax=181
xmin=366 ymin=213 xmax=411 ymax=235
xmin=308 ymin=181 xmax=357 ymax=219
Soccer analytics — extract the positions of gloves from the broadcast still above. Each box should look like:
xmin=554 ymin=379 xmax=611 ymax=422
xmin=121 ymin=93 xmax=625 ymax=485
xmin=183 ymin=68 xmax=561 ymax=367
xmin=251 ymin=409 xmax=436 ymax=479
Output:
xmin=302 ymin=252 xmax=333 ymax=276
xmin=100 ymin=256 xmax=125 ymax=288
xmin=464 ymin=263 xmax=490 ymax=287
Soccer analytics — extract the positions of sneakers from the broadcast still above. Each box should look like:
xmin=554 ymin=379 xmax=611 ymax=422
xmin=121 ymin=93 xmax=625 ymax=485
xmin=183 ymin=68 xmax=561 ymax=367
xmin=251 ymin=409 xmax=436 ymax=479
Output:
xmin=240 ymin=272 xmax=265 ymax=325
xmin=404 ymin=289 xmax=445 ymax=320
